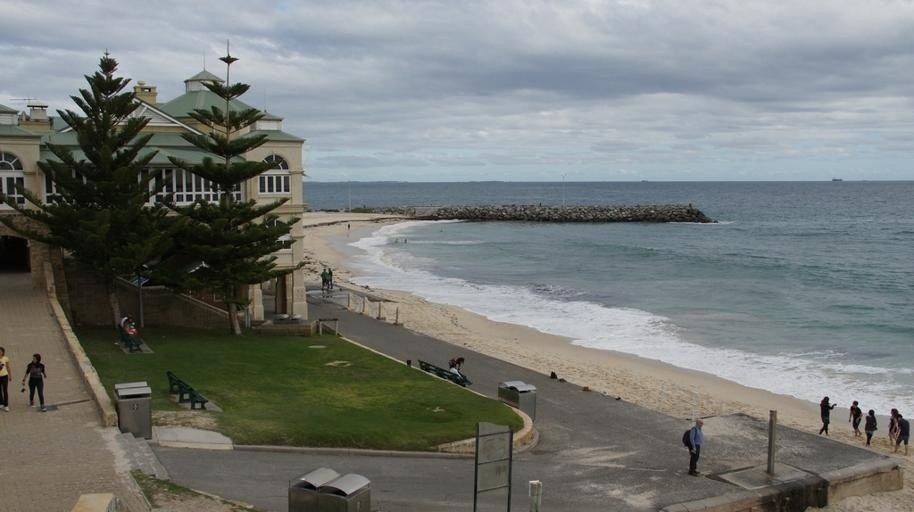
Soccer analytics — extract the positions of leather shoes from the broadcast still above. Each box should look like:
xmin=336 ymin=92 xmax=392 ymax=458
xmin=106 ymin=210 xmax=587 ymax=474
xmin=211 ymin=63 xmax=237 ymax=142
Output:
xmin=689 ymin=469 xmax=700 ymax=473
xmin=688 ymin=471 xmax=697 ymax=476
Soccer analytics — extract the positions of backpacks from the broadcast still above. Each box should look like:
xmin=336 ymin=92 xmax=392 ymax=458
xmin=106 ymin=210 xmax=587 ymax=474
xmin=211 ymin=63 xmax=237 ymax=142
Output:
xmin=683 ymin=427 xmax=697 ymax=447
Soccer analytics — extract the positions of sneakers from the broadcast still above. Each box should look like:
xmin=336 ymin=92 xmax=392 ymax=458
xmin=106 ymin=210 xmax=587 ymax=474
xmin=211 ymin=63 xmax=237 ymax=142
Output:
xmin=0 ymin=403 xmax=47 ymax=413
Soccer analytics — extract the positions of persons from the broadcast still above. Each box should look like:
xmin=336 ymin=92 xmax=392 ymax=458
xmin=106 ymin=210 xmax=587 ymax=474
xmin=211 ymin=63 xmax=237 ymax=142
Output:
xmin=686 ymin=417 xmax=703 ymax=476
xmin=819 ymin=395 xmax=837 ymax=437
xmin=120 ymin=312 xmax=141 ymax=347
xmin=893 ymin=414 xmax=909 ymax=456
xmin=326 ymin=268 xmax=333 ymax=290
xmin=320 ymin=268 xmax=329 ymax=290
xmin=447 ymin=354 xmax=464 ymax=371
xmin=863 ymin=408 xmax=878 ymax=446
xmin=887 ymin=408 xmax=899 ymax=448
xmin=21 ymin=353 xmax=48 ymax=412
xmin=0 ymin=346 xmax=11 ymax=411
xmin=847 ymin=400 xmax=863 ymax=438
xmin=448 ymin=363 xmax=472 ymax=387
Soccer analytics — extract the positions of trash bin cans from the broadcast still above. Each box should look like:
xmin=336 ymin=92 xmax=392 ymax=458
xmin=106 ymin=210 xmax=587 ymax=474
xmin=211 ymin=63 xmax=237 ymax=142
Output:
xmin=288 ymin=467 xmax=372 ymax=512
xmin=113 ymin=381 xmax=152 ymax=439
xmin=498 ymin=381 xmax=536 ymax=423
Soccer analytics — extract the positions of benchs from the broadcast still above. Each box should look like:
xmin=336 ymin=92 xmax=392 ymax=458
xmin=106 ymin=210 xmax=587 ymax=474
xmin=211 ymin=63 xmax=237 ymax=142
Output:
xmin=417 ymin=358 xmax=473 ymax=389
xmin=166 ymin=370 xmax=209 ymax=412
xmin=118 ymin=324 xmax=144 ymax=354
